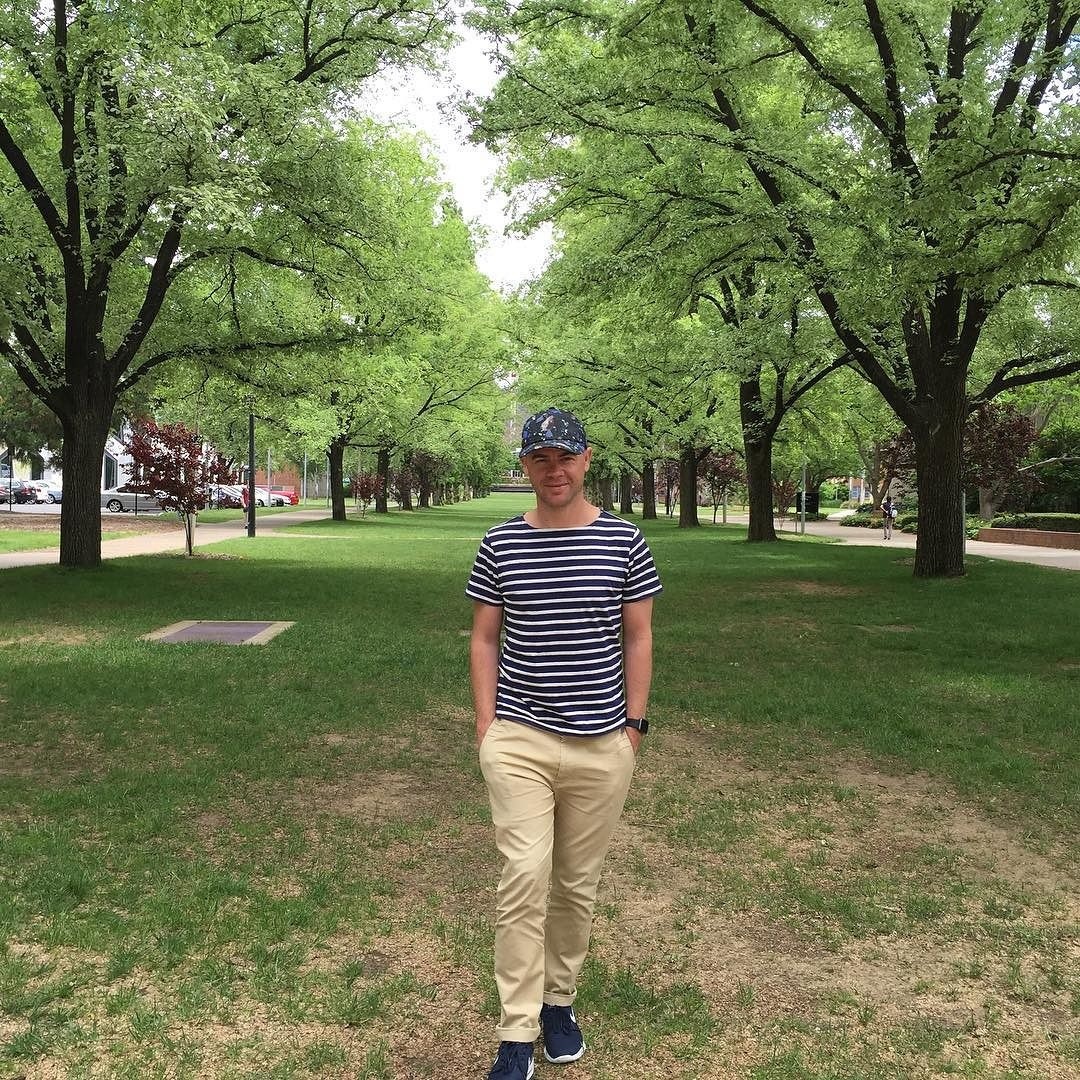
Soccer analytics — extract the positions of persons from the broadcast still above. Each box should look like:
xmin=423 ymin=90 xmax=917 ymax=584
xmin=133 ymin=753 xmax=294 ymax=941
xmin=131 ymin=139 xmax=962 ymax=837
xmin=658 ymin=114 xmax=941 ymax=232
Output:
xmin=465 ymin=408 xmax=663 ymax=1080
xmin=241 ymin=480 xmax=249 ymax=529
xmin=880 ymin=496 xmax=895 ymax=540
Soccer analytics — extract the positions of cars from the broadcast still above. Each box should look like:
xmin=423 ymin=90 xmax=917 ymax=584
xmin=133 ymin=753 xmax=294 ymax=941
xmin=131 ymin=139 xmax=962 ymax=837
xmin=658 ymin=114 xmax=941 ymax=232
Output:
xmin=101 ymin=481 xmax=300 ymax=513
xmin=0 ymin=480 xmax=62 ymax=505
xmin=0 ymin=464 xmax=12 ymax=478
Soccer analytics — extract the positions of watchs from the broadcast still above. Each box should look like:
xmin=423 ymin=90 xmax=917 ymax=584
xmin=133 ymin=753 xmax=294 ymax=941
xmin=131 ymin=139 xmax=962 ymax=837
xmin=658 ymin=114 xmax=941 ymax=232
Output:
xmin=624 ymin=718 xmax=649 ymax=735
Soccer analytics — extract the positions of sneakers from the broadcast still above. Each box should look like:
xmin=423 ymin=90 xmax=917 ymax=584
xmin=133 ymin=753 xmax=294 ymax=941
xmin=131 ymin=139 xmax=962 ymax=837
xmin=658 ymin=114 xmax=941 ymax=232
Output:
xmin=539 ymin=1002 xmax=585 ymax=1063
xmin=487 ymin=1043 xmax=534 ymax=1080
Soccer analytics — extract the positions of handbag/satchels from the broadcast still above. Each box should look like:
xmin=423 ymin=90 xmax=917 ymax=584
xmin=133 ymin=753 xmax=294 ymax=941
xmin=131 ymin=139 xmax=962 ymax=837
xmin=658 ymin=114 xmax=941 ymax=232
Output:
xmin=890 ymin=508 xmax=897 ymax=518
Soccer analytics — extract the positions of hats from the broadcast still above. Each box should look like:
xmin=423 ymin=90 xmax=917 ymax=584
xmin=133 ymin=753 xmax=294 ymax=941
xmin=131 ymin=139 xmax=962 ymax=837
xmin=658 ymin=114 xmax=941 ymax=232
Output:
xmin=519 ymin=407 xmax=587 ymax=454
